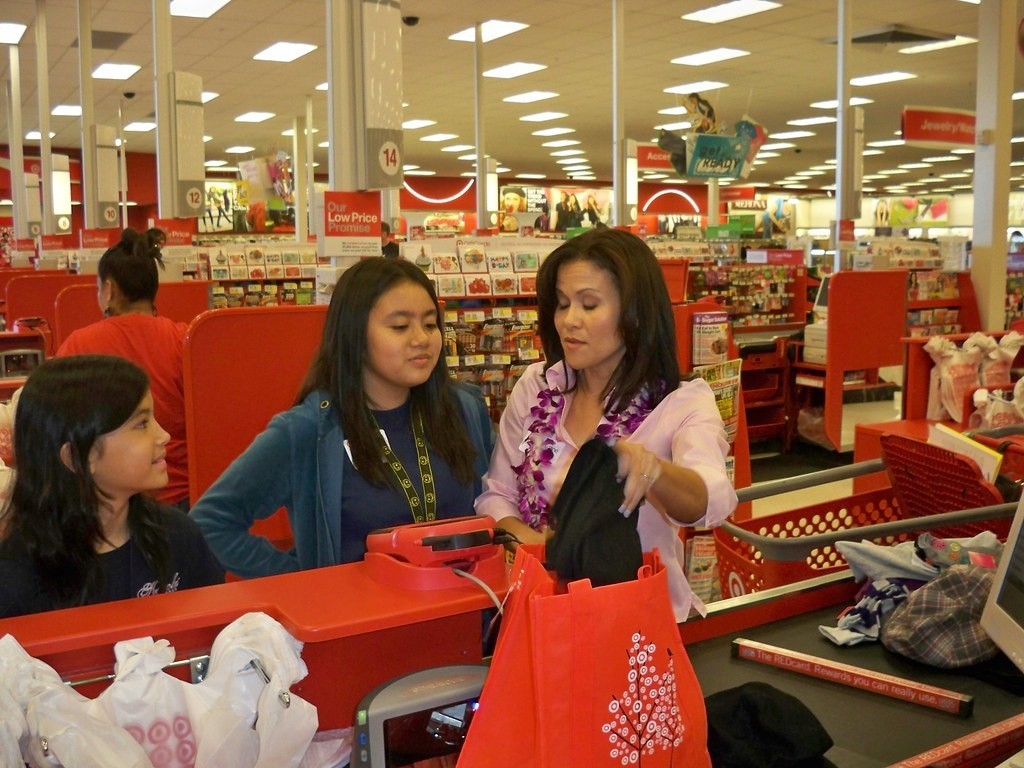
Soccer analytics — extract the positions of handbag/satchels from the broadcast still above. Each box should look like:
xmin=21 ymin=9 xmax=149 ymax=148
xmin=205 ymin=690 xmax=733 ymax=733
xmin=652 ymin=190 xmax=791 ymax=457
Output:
xmin=0 ymin=611 xmax=319 ymax=768
xmin=456 ymin=544 xmax=713 ymax=768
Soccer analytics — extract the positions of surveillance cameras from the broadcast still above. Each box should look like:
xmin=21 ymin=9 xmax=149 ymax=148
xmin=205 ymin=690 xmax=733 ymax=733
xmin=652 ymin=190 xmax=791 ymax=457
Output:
xmin=402 ymin=17 xmax=419 ymax=27
xmin=795 ymin=149 xmax=801 ymax=153
xmin=124 ymin=92 xmax=136 ymax=98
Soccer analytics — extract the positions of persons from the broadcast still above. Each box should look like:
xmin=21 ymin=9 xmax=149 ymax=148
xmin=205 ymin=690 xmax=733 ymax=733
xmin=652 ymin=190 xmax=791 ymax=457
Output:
xmin=203 ymin=186 xmax=233 ymax=233
xmin=188 ymin=256 xmax=498 ymax=579
xmin=53 ymin=227 xmax=192 ymax=515
xmin=0 ymin=355 xmax=223 ymax=618
xmin=381 ymin=221 xmax=399 ymax=258
xmin=908 ymin=272 xmax=920 ymax=301
xmin=553 ymin=191 xmax=609 ymax=230
xmin=762 ymin=198 xmax=791 ymax=239
xmin=500 ymin=187 xmax=527 ymax=213
xmin=474 ymin=225 xmax=739 ymax=624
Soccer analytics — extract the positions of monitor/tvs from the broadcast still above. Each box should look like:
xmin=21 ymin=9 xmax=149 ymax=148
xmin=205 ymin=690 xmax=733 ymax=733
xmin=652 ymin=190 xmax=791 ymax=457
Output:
xmin=813 ymin=276 xmax=830 ymax=312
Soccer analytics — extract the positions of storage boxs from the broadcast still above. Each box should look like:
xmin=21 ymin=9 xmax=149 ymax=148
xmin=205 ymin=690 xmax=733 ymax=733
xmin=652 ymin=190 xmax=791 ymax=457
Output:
xmin=208 ymin=240 xmax=564 ymax=296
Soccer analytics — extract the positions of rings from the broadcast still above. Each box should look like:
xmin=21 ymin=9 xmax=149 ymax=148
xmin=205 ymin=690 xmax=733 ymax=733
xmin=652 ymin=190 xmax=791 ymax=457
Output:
xmin=641 ymin=473 xmax=650 ymax=481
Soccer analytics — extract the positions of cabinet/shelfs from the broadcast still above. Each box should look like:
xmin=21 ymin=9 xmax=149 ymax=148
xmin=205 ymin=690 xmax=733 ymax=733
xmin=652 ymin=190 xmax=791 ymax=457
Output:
xmin=183 ymin=259 xmax=1024 ymax=609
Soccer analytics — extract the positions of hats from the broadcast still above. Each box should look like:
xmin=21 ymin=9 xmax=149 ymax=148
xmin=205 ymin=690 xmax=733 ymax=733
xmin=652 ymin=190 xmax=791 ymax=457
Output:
xmin=882 ymin=563 xmax=1001 ymax=667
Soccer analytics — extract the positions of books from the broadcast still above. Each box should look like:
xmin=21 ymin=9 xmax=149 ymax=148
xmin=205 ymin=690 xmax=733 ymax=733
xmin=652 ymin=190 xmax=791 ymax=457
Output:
xmin=927 ymin=423 xmax=1003 ymax=485
xmin=908 ymin=272 xmax=961 ymax=338
xmin=687 ymin=311 xmax=744 ymax=606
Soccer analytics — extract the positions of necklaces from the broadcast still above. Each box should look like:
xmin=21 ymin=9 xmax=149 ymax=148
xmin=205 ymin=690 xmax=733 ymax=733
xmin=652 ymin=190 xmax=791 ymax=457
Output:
xmin=510 ymin=377 xmax=669 ymax=530
